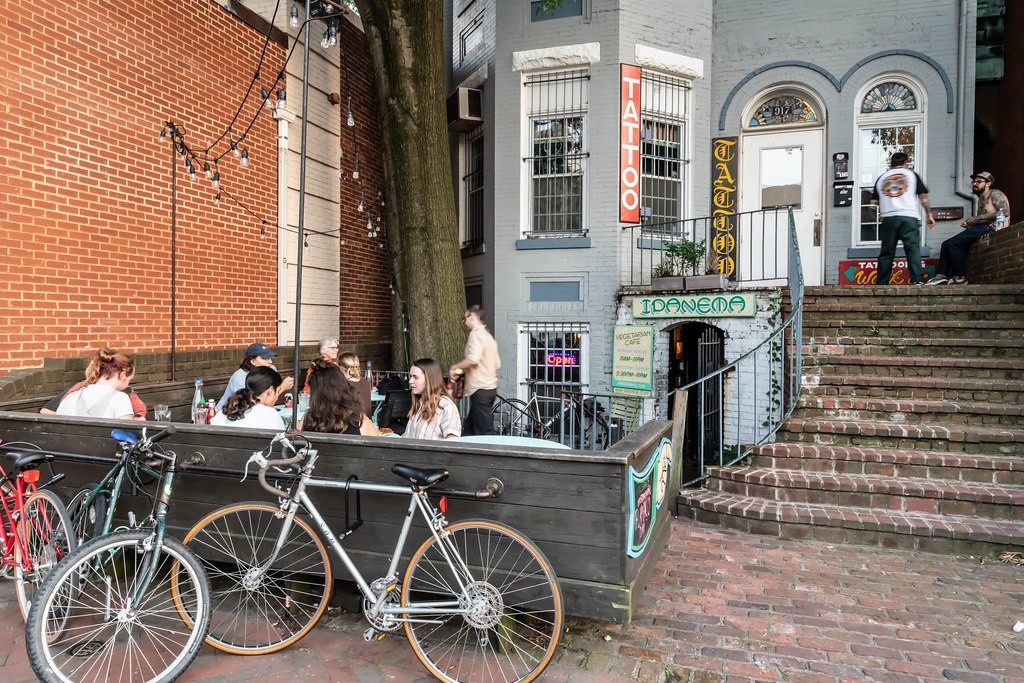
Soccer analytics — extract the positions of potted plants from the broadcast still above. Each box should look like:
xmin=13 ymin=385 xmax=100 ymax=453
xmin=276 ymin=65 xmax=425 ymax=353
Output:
xmin=652 ymin=235 xmax=728 ymax=290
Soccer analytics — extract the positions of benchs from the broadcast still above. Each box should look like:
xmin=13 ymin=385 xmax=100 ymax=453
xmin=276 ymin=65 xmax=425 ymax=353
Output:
xmin=0 ymin=367 xmax=671 ymax=657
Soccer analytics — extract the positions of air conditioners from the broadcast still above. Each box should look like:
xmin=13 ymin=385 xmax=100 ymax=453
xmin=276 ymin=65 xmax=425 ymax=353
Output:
xmin=446 ymin=87 xmax=482 ymax=131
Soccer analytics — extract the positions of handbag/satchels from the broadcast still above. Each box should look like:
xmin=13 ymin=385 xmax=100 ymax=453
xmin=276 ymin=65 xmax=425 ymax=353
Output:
xmin=377 ymin=376 xmax=404 ymax=396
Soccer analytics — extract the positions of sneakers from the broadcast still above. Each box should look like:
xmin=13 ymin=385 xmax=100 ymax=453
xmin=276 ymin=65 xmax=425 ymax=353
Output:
xmin=925 ymin=276 xmax=948 ymax=285
xmin=945 ymin=275 xmax=969 ymax=285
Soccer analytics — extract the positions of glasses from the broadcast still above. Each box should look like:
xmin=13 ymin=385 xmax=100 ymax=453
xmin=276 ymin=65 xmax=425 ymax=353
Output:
xmin=327 ymin=347 xmax=339 ymax=351
xmin=971 ymin=179 xmax=985 ymax=185
xmin=462 ymin=314 xmax=472 ymax=322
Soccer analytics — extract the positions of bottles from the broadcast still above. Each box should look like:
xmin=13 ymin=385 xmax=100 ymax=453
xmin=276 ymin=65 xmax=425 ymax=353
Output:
xmin=191 ymin=378 xmax=216 ymax=425
xmin=364 ymin=361 xmax=373 ymax=391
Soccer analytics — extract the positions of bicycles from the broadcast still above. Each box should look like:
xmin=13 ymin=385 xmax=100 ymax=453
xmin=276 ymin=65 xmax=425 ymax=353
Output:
xmin=0 ymin=426 xmax=213 ymax=682
xmin=169 ymin=420 xmax=565 ymax=683
xmin=491 ymin=377 xmax=608 ymax=452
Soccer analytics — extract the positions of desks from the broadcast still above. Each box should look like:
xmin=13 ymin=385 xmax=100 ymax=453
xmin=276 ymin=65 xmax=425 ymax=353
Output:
xmin=273 ymin=404 xmax=307 ymax=431
xmin=368 ymin=388 xmax=386 ymax=424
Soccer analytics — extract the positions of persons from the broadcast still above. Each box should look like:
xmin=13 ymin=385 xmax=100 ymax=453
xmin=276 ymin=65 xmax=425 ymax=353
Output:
xmin=870 ymin=153 xmax=935 ymax=285
xmin=449 ymin=305 xmax=501 ymax=436
xmin=214 ymin=342 xmax=294 ymax=413
xmin=303 ymin=338 xmax=340 ymax=393
xmin=925 ymin=171 xmax=1010 ymax=284
xmin=402 ymin=358 xmax=462 ymax=440
xmin=296 ymin=366 xmax=376 ymax=436
xmin=209 ymin=366 xmax=286 ymax=430
xmin=338 ymin=352 xmax=371 ymax=419
xmin=39 ymin=346 xmax=147 ymax=420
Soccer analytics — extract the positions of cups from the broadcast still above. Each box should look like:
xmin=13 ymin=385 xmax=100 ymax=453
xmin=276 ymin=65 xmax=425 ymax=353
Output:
xmin=298 ymin=392 xmax=309 ymax=412
xmin=157 ymin=411 xmax=171 ymax=423
xmin=154 ymin=405 xmax=168 ymax=420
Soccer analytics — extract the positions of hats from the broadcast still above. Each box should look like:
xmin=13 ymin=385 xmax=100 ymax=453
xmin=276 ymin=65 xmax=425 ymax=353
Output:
xmin=246 ymin=343 xmax=279 ymax=359
xmin=970 ymin=171 xmax=994 ymax=187
